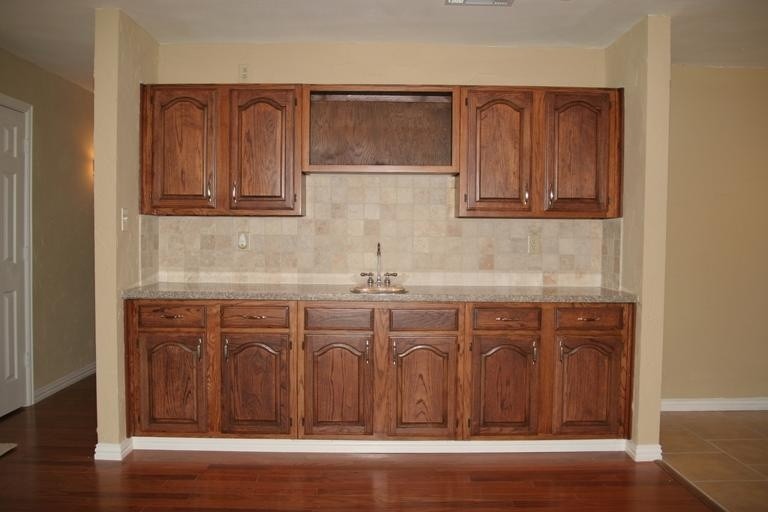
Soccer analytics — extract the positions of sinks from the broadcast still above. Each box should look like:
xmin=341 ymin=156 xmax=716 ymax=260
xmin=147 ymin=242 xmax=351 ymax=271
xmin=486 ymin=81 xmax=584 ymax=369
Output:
xmin=350 ymin=285 xmax=409 ymax=295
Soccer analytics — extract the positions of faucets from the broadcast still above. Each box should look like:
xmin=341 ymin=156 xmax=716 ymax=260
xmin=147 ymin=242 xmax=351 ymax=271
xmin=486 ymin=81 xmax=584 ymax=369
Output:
xmin=375 ymin=242 xmax=383 ymax=284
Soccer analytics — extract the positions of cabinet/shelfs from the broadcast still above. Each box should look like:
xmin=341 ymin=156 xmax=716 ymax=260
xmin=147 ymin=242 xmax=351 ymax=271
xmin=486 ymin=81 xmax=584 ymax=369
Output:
xmin=298 ymin=302 xmax=465 ymax=439
xmin=301 ymin=82 xmax=460 ymax=176
xmin=139 ymin=79 xmax=306 ymax=219
xmin=452 ymin=84 xmax=625 ymax=221
xmin=122 ymin=298 xmax=298 ymax=444
xmin=464 ymin=301 xmax=637 ymax=441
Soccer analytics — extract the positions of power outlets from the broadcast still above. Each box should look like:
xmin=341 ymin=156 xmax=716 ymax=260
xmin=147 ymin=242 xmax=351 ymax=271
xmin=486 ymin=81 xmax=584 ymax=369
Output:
xmin=527 ymin=235 xmax=542 ymax=256
